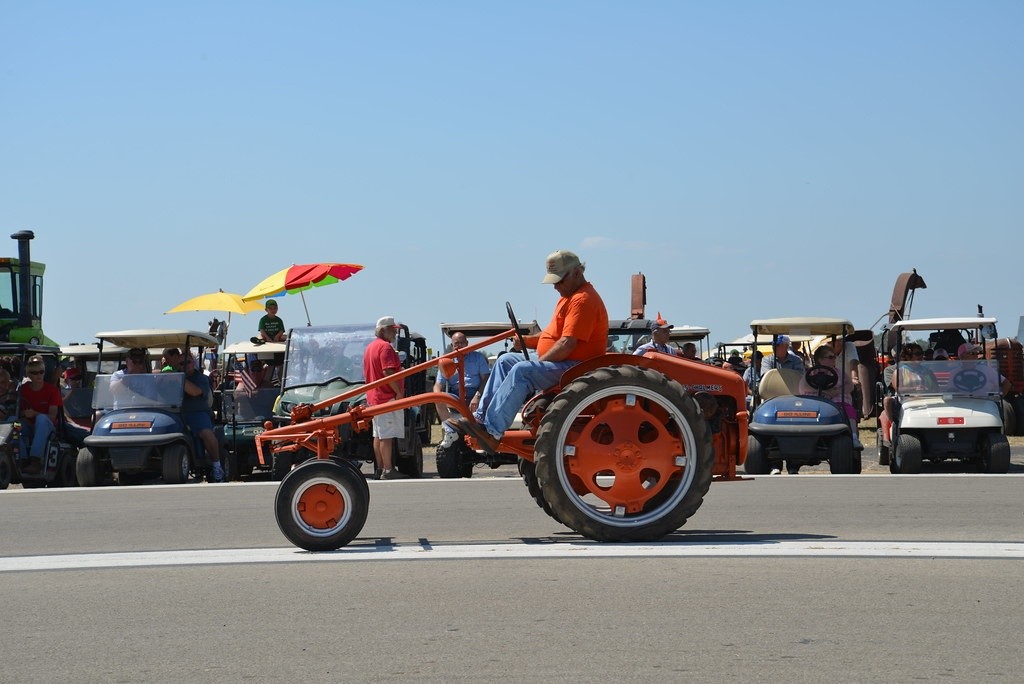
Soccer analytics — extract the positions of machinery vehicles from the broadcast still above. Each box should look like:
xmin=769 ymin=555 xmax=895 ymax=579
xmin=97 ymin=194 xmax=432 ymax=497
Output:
xmin=0 ymin=257 xmax=61 ymax=347
xmin=255 ymin=302 xmax=749 ymax=552
xmin=976 ymin=337 xmax=1024 ymax=437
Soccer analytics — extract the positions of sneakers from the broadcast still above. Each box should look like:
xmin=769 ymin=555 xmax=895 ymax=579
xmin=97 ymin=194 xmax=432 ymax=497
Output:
xmin=852 ymin=439 xmax=864 ymax=451
xmin=440 ymin=431 xmax=459 ymax=449
xmin=375 ymin=468 xmax=382 ymax=480
xmin=380 ymin=469 xmax=410 ymax=479
xmin=212 ymin=461 xmax=225 ymax=481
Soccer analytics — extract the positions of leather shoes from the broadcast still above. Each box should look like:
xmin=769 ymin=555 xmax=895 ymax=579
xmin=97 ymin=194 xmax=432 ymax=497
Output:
xmin=446 ymin=417 xmax=476 ymax=435
xmin=458 ymin=415 xmax=500 ymax=457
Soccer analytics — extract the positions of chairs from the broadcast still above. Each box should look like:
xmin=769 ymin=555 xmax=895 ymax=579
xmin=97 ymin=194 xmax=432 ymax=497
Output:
xmin=760 ymin=369 xmax=802 ymax=407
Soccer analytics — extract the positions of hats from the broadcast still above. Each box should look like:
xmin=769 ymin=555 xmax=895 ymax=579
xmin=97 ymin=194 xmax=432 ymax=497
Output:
xmin=543 ymin=251 xmax=581 ymax=284
xmin=891 ymin=344 xmax=905 ymax=356
xmin=63 ymin=368 xmax=78 ymax=379
xmin=127 ymin=348 xmax=144 ymax=359
xmin=770 ymin=335 xmax=790 ymax=346
xmin=957 ymin=343 xmax=983 ymax=357
xmin=376 ymin=316 xmax=401 ymax=329
xmin=265 ymin=299 xmax=278 ymax=307
xmin=250 ymin=359 xmax=263 ymax=369
xmin=933 ymin=348 xmax=948 ymax=360
xmin=651 ymin=318 xmax=674 ymax=331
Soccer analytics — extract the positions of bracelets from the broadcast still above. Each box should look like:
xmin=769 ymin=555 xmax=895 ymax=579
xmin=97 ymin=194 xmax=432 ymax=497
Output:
xmin=476 ymin=392 xmax=481 ymax=397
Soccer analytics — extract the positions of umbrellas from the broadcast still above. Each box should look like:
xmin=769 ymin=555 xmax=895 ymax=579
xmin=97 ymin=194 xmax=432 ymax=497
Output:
xmin=163 ymin=289 xmax=266 ymax=325
xmin=242 ymin=262 xmax=366 ymax=326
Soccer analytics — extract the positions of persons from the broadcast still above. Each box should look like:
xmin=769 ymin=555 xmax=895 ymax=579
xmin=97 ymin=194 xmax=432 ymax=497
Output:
xmin=0 ymin=346 xmax=348 ymax=481
xmin=250 ymin=300 xmax=287 ymax=345
xmin=363 ymin=317 xmax=410 ymax=480
xmin=633 ymin=320 xmax=1012 ymax=474
xmin=444 ymin=250 xmax=609 ymax=456
xmin=433 ymin=332 xmax=492 ymax=454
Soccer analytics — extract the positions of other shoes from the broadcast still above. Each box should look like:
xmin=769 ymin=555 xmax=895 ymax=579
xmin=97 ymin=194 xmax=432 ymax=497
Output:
xmin=22 ymin=463 xmax=40 ymax=473
xmin=16 ymin=461 xmax=28 ymax=471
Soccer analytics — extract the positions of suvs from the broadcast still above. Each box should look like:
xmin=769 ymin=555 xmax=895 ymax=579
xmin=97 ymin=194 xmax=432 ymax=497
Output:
xmin=272 ymin=323 xmax=427 ymax=481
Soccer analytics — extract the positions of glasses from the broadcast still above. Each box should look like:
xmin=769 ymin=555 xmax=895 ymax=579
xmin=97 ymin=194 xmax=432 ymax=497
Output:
xmin=29 ymin=370 xmax=45 ymax=375
xmin=821 ymin=355 xmax=836 ymax=359
xmin=177 ymin=359 xmax=193 ymax=366
xmin=249 ymin=367 xmax=263 ymax=372
xmin=912 ymin=351 xmax=924 ymax=355
xmin=129 ymin=359 xmax=144 ymax=364
xmin=654 ymin=330 xmax=673 ymax=334
xmin=963 ymin=351 xmax=979 ymax=355
xmin=68 ymin=375 xmax=82 ymax=380
